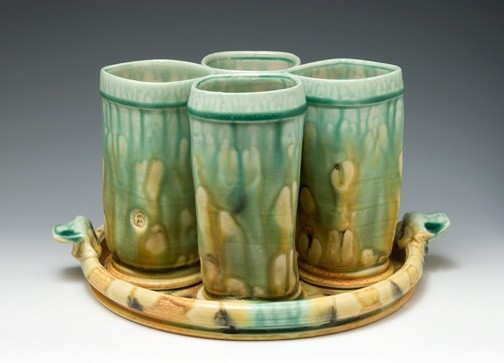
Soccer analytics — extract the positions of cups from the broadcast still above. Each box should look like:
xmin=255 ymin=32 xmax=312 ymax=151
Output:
xmin=98 ymin=57 xmax=216 ymax=292
xmin=200 ymin=49 xmax=302 ymax=75
xmin=283 ymin=57 xmax=405 ymax=290
xmin=185 ymin=71 xmax=308 ymax=299
